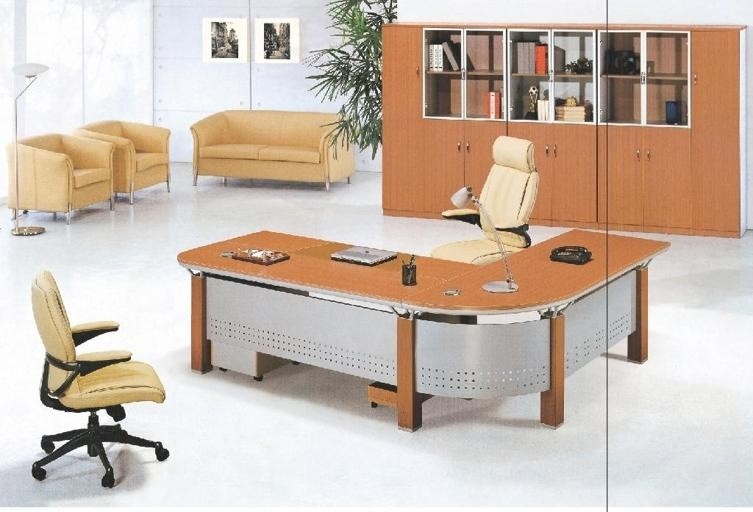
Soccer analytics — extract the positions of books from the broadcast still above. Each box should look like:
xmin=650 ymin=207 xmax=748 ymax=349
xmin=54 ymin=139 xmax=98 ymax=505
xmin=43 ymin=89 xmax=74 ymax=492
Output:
xmin=424 ymin=40 xmax=592 ymax=122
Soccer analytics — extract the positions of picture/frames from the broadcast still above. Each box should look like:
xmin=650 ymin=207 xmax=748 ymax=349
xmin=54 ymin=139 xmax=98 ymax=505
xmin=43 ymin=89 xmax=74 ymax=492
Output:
xmin=199 ymin=18 xmax=300 ymax=67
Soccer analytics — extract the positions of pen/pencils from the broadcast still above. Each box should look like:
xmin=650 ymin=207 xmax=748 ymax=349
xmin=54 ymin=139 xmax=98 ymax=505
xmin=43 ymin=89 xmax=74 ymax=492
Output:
xmin=401 ymin=254 xmax=415 ymax=284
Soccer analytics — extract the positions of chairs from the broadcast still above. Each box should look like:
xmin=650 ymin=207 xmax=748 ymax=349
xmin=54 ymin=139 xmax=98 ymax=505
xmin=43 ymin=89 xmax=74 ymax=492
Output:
xmin=23 ymin=272 xmax=169 ymax=486
xmin=8 ymin=121 xmax=170 ymax=225
xmin=430 ymin=135 xmax=540 ymax=269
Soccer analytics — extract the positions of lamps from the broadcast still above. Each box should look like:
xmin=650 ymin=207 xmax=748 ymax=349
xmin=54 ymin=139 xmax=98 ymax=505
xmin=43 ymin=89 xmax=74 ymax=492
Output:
xmin=446 ymin=181 xmax=517 ymax=292
xmin=7 ymin=61 xmax=51 ymax=237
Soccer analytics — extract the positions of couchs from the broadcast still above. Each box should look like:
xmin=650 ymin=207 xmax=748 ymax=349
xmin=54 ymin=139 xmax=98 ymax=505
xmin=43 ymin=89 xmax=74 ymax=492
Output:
xmin=188 ymin=108 xmax=355 ymax=192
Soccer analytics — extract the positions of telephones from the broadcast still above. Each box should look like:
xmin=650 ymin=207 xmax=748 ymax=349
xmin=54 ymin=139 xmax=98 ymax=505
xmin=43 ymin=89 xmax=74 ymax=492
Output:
xmin=549 ymin=246 xmax=592 ymax=265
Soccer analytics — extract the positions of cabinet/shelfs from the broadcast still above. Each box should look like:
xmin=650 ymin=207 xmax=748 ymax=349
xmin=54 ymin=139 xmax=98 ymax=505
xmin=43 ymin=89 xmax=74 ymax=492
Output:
xmin=424 ymin=25 xmax=507 ymax=219
xmin=505 ymin=24 xmax=597 ymax=228
xmin=597 ymin=26 xmax=693 ymax=233
xmin=690 ymin=29 xmax=741 ymax=234
xmin=382 ymin=23 xmax=424 ymax=220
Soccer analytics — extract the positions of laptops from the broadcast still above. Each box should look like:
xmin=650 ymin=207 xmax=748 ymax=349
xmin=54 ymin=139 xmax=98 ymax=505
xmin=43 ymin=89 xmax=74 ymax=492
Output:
xmin=330 ymin=245 xmax=398 ymax=265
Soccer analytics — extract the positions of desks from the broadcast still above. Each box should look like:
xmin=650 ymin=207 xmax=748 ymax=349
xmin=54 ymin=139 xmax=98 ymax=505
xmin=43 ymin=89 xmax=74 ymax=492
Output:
xmin=177 ymin=230 xmax=668 ymax=433
